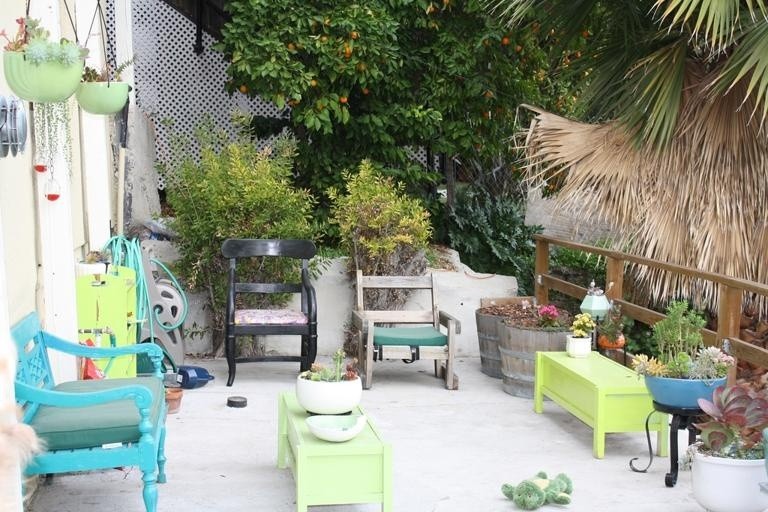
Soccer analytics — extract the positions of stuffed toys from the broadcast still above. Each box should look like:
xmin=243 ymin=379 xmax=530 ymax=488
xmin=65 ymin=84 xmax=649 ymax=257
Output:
xmin=501 ymin=469 xmax=574 ymax=510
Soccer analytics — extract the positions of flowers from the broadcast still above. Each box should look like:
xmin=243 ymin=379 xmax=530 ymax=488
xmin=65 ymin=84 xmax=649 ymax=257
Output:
xmin=535 ymin=304 xmax=561 ymax=330
xmin=568 ymin=312 xmax=595 ymax=337
xmin=521 ymin=299 xmax=529 ymax=309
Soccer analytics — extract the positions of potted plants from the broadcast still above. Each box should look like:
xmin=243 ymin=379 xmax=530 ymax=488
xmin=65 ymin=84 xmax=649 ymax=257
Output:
xmin=579 ymin=279 xmax=614 ymax=321
xmin=690 ymin=383 xmax=767 ymax=512
xmin=595 ymin=305 xmax=626 ymax=349
xmin=630 ymin=298 xmax=734 ymax=409
xmin=0 ymin=0 xmax=138 ymax=115
xmin=295 ymin=347 xmax=362 ymax=415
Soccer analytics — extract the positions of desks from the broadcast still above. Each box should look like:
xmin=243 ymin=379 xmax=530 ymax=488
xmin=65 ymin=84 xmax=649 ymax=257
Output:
xmin=629 ymin=400 xmax=710 ymax=487
xmin=532 ymin=349 xmax=668 ymax=459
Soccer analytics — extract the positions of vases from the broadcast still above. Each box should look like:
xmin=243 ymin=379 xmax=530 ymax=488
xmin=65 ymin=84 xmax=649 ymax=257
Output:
xmin=475 ymin=308 xmax=503 ymax=379
xmin=496 ymin=310 xmax=574 ymax=401
xmin=565 ymin=335 xmax=591 ymax=358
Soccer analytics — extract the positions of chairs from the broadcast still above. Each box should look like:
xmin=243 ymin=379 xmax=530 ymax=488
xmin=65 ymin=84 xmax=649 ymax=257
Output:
xmin=352 ymin=269 xmax=461 ymax=391
xmin=220 ymin=239 xmax=318 ymax=387
xmin=8 ymin=312 xmax=169 ymax=512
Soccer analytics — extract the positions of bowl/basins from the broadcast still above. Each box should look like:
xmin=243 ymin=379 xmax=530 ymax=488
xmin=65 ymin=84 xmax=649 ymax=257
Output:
xmin=306 ymin=413 xmax=366 ymax=442
